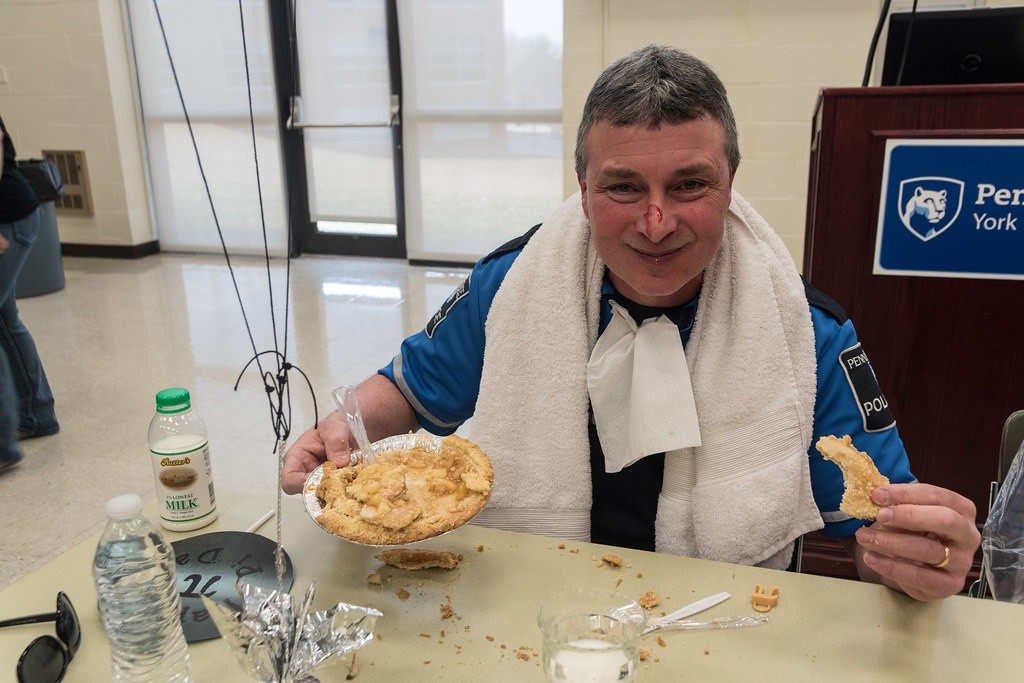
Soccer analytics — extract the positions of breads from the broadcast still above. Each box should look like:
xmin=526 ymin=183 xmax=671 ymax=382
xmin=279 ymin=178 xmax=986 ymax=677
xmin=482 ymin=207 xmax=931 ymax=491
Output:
xmin=815 ymin=434 xmax=890 ymax=520
xmin=314 ymin=434 xmax=496 ymax=544
xmin=374 ymin=549 xmax=460 ymax=571
xmin=640 ymin=591 xmax=660 ymax=609
xmin=602 ymin=555 xmax=621 ymax=566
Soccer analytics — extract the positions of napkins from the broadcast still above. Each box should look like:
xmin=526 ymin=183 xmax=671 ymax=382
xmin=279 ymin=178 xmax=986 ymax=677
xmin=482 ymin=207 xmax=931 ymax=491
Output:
xmin=585 ymin=299 xmax=703 ymax=474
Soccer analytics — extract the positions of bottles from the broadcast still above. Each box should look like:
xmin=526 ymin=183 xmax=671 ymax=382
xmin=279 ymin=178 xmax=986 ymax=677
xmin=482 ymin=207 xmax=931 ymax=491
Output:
xmin=92 ymin=494 xmax=195 ymax=683
xmin=147 ymin=387 xmax=219 ymax=532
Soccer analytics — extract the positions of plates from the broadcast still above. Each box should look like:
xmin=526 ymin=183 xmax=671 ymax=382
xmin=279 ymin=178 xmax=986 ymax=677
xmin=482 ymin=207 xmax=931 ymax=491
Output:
xmin=302 ymin=434 xmax=494 ymax=548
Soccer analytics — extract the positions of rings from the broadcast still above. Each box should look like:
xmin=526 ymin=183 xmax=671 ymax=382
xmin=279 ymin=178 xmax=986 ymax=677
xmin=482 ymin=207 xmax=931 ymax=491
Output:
xmin=933 ymin=543 xmax=951 ymax=569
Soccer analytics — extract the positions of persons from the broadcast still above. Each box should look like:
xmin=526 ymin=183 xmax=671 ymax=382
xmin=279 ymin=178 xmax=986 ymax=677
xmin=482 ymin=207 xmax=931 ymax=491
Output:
xmin=0 ymin=113 xmax=60 ymax=473
xmin=280 ymin=39 xmax=984 ymax=606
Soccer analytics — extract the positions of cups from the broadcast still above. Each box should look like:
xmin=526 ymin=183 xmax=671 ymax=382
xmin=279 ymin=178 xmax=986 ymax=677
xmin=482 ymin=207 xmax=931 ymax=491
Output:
xmin=537 ymin=585 xmax=647 ymax=683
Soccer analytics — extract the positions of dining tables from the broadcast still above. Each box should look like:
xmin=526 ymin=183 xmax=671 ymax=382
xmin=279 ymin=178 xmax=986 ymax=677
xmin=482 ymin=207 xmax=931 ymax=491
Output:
xmin=2 ymin=482 xmax=1024 ymax=683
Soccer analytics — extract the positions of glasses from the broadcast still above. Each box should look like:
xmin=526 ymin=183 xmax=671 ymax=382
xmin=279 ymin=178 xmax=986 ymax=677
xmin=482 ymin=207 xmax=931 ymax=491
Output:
xmin=0 ymin=591 xmax=82 ymax=683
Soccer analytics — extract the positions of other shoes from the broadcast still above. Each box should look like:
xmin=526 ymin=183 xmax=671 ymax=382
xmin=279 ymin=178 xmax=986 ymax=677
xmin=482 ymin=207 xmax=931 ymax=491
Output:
xmin=0 ymin=407 xmax=59 ymax=439
xmin=0 ymin=449 xmax=23 ymax=475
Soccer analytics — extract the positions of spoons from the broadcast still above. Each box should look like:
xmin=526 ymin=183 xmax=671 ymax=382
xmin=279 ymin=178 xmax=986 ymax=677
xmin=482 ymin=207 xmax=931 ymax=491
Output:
xmin=330 ymin=383 xmax=407 ymax=502
